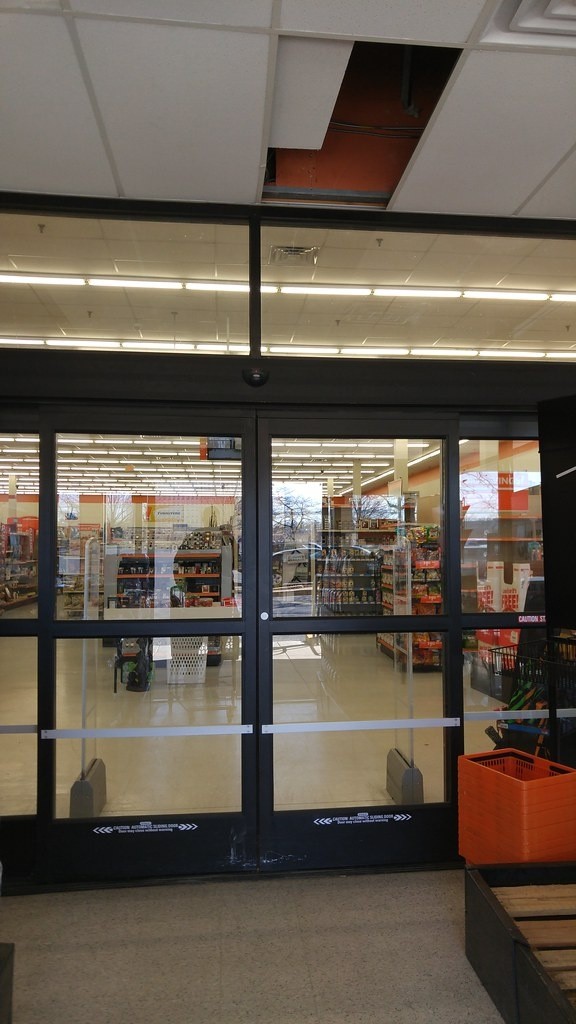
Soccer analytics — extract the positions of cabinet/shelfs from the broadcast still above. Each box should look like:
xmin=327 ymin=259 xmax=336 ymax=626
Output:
xmin=0 ymin=495 xmax=542 ymax=674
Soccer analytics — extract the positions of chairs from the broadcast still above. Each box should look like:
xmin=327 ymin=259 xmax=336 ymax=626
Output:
xmin=113 ymin=637 xmax=152 ymax=693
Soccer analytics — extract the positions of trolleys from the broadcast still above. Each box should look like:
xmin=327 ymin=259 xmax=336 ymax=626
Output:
xmin=489 ymin=639 xmax=576 ymax=767
